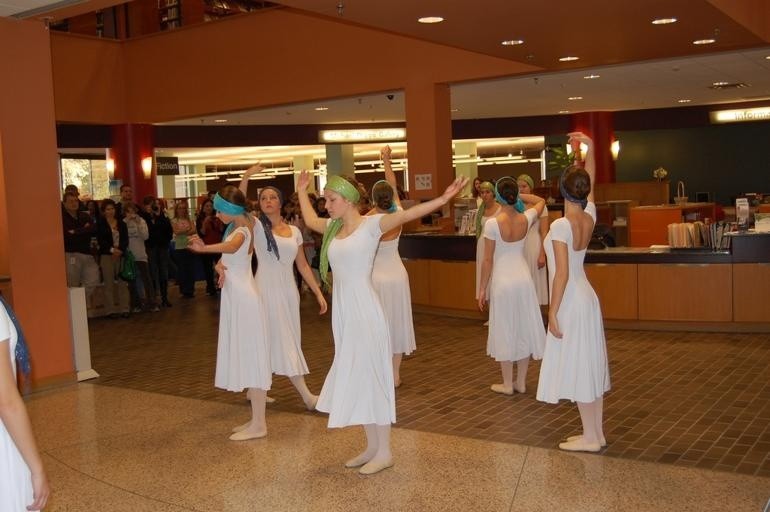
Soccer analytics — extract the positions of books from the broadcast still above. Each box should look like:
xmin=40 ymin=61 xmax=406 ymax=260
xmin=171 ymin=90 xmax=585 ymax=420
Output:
xmin=667 ymin=223 xmax=733 ymax=250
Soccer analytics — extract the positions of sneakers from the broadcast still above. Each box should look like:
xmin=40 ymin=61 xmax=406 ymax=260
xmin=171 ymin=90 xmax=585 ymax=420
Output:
xmin=558 ymin=434 xmax=607 ymax=452
xmin=491 ymin=381 xmax=527 ymax=395
xmin=229 ymin=379 xmax=403 ymax=475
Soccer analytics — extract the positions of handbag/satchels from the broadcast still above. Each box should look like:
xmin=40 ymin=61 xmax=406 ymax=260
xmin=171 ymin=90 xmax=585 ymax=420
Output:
xmin=119 ymin=250 xmax=137 ymax=281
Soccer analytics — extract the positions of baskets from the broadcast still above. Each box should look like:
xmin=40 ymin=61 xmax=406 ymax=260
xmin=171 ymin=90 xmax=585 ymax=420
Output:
xmin=673 ymin=181 xmax=688 ymax=205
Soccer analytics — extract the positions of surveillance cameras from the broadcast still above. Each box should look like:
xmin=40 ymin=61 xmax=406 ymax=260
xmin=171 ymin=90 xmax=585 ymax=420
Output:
xmin=387 ymin=95 xmax=394 ymax=99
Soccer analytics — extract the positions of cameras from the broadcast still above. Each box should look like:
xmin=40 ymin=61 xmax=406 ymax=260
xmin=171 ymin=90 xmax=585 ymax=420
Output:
xmin=152 ymin=205 xmax=158 ymax=212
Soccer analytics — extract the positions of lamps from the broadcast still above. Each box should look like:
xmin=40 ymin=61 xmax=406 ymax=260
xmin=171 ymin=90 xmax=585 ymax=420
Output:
xmin=353 ymin=141 xmax=409 ymax=175
xmin=452 ymin=150 xmax=542 ymax=166
xmin=170 ymin=143 xmax=294 ymax=182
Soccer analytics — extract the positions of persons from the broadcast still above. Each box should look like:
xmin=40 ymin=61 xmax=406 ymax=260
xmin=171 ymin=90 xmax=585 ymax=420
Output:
xmin=0 ymin=292 xmax=51 ymax=511
xmin=296 ymin=169 xmax=472 ymax=475
xmin=363 ymin=145 xmax=417 ymax=388
xmin=473 ymin=175 xmax=547 ymax=325
xmin=62 ymin=183 xmax=225 ymax=321
xmin=476 ymin=177 xmax=545 ymax=396
xmin=537 ymin=132 xmax=610 ymax=453
xmin=357 ymin=183 xmax=373 ymax=214
xmin=238 ymin=160 xmax=329 ymax=411
xmin=396 ymin=185 xmax=422 ymax=230
xmin=185 ymin=185 xmax=273 ymax=441
xmin=220 ymin=180 xmax=330 ymax=297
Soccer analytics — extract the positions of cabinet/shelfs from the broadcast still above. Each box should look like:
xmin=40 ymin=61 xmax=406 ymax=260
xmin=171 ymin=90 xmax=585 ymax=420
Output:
xmin=627 ymin=202 xmax=716 ymax=248
